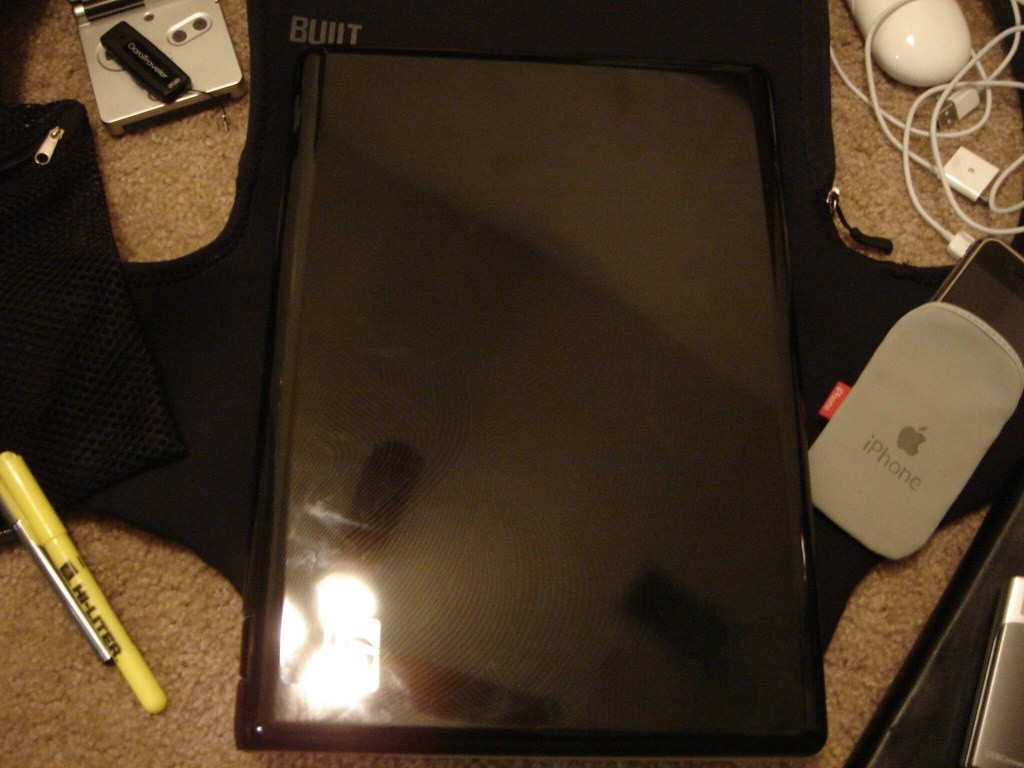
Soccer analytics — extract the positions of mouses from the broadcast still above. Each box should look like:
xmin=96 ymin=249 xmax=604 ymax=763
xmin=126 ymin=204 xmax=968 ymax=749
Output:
xmin=846 ymin=0 xmax=972 ymax=88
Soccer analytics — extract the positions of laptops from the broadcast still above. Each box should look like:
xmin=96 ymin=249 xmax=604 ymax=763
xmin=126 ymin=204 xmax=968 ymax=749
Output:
xmin=234 ymin=50 xmax=828 ymax=761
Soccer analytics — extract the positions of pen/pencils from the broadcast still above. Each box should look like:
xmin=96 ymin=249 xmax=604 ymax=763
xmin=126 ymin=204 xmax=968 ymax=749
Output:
xmin=0 ymin=453 xmax=165 ymax=713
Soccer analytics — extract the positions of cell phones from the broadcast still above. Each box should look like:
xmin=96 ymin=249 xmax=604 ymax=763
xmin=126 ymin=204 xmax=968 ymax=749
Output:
xmin=959 ymin=576 xmax=1024 ymax=768
xmin=925 ymin=237 xmax=1024 ymax=369
